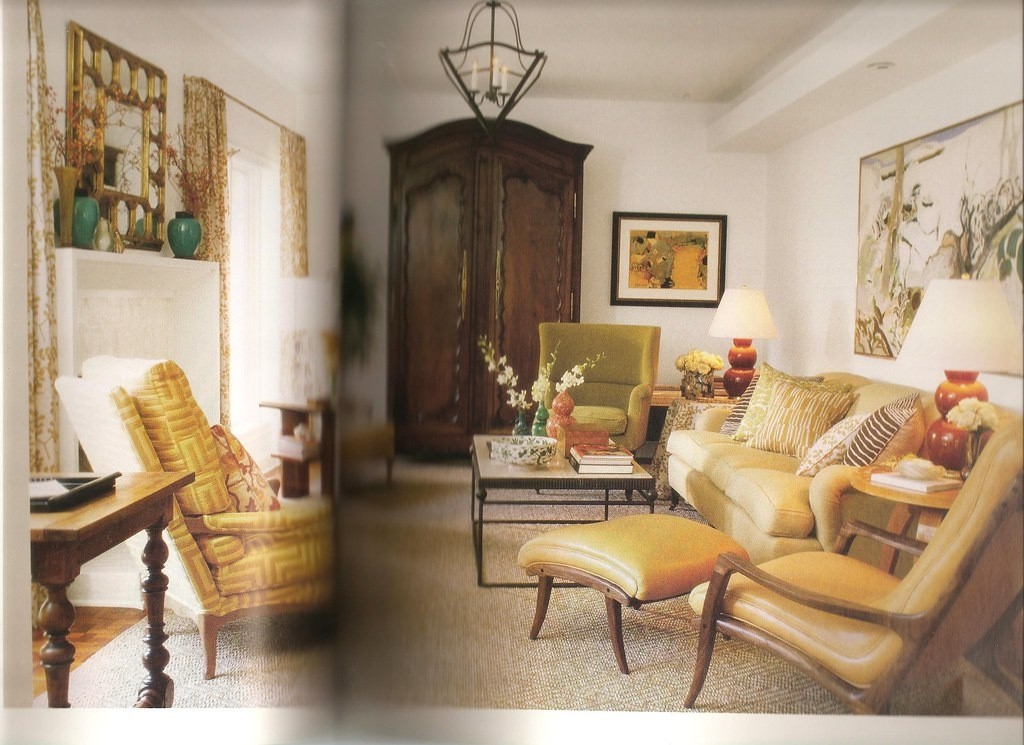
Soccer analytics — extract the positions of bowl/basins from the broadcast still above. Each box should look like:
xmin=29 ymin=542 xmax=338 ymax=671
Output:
xmin=491 ymin=435 xmax=558 ymax=465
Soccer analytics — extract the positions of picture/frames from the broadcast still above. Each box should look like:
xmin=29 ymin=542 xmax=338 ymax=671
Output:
xmin=609 ymin=211 xmax=728 ymax=308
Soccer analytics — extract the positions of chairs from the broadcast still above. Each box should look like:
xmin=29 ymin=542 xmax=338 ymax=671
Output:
xmin=535 ymin=322 xmax=660 ymax=453
xmin=109 ymin=387 xmax=340 ymax=680
xmin=683 ymin=413 xmax=1023 ymax=716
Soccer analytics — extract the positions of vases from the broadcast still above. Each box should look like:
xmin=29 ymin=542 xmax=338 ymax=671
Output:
xmin=511 ymin=409 xmax=530 ymax=436
xmin=50 ymin=189 xmax=102 ymax=248
xmin=545 ymin=390 xmax=578 ymax=438
xmin=683 ymin=368 xmax=714 ymax=401
xmin=926 ymin=370 xmax=988 ymax=471
xmin=530 ymin=400 xmax=548 ymax=436
xmin=959 ymin=426 xmax=984 ymax=480
xmin=168 ymin=211 xmax=202 ymax=259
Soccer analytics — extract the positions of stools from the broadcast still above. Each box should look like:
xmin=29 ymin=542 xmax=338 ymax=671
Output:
xmin=515 ymin=510 xmax=751 ymax=674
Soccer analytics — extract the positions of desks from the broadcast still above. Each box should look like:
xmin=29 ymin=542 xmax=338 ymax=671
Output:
xmin=467 ymin=434 xmax=655 ymax=588
xmin=848 ymin=462 xmax=967 ymax=577
xmin=26 ymin=469 xmax=195 ymax=709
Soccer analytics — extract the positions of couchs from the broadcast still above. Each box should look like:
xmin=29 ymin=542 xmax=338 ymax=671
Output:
xmin=666 ymin=370 xmax=937 ymax=567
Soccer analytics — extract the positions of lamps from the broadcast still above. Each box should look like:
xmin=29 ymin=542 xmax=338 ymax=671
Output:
xmin=706 ymin=283 xmax=777 ymax=400
xmin=439 ymin=0 xmax=548 ymax=135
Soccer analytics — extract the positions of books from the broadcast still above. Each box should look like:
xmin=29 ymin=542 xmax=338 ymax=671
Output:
xmin=871 ymin=474 xmax=963 ymax=492
xmin=571 ymin=444 xmax=634 ymax=473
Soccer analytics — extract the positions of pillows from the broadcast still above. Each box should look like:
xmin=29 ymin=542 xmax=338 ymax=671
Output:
xmin=207 ymin=424 xmax=279 ymax=512
xmin=794 ymin=413 xmax=870 ymax=477
xmin=730 ymin=363 xmax=854 ymax=446
xmin=131 ymin=362 xmax=232 ymax=515
xmin=840 ymin=390 xmax=926 ymax=464
xmin=740 ymin=380 xmax=855 ymax=462
xmin=717 ymin=366 xmax=824 ymax=435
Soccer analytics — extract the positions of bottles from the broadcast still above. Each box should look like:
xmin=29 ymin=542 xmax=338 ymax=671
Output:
xmin=167 ymin=211 xmax=202 ymax=259
xmin=96 ymin=216 xmax=111 ymax=250
xmin=54 ymin=189 xmax=99 ymax=249
xmin=294 ymin=420 xmax=309 ymax=444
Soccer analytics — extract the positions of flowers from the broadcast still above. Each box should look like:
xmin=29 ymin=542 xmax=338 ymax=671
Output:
xmin=555 ymin=352 xmax=605 ymax=394
xmin=674 ymin=349 xmax=724 ymax=375
xmin=34 ymin=83 xmax=135 ymax=196
xmin=477 ymin=334 xmax=532 ymax=410
xmin=151 ymin=120 xmax=241 ymax=215
xmin=944 ymin=397 xmax=1001 ymax=434
xmin=530 ymin=339 xmax=562 ymax=402
